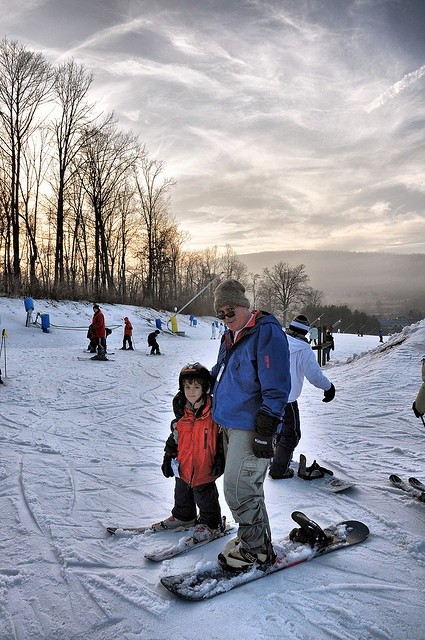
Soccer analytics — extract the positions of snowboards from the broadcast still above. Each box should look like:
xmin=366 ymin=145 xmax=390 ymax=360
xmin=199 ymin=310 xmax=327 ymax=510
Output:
xmin=161 ymin=520 xmax=368 ymax=600
xmin=289 ymin=459 xmax=356 ymax=492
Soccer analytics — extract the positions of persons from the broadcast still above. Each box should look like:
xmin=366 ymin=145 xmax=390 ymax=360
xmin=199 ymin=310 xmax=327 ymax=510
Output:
xmin=152 ymin=362 xmax=225 ymax=547
xmin=379 ymin=330 xmax=383 ymax=342
xmin=90 ymin=305 xmax=107 ymax=360
xmin=285 ymin=321 xmax=289 ymax=331
xmin=120 ymin=316 xmax=133 ymax=350
xmin=309 ymin=325 xmax=318 ymax=345
xmin=325 ymin=331 xmax=335 ymax=362
xmin=87 ymin=324 xmax=112 ymax=353
xmin=210 ymin=323 xmax=216 ymax=340
xmin=208 ymin=278 xmax=292 ymax=572
xmin=412 ymin=356 xmax=425 ymax=419
xmin=148 ymin=329 xmax=161 ymax=355
xmin=328 ymin=325 xmax=334 ymax=335
xmin=218 ymin=323 xmax=223 ymax=339
xmin=270 ymin=314 xmax=336 ymax=479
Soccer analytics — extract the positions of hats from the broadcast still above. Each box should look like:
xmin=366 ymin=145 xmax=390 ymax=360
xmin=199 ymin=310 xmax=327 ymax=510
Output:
xmin=214 ymin=279 xmax=250 ymax=313
xmin=289 ymin=315 xmax=311 ymax=336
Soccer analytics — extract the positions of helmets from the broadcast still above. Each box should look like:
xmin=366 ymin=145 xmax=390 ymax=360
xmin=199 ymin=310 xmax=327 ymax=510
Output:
xmin=179 ymin=362 xmax=210 ymax=393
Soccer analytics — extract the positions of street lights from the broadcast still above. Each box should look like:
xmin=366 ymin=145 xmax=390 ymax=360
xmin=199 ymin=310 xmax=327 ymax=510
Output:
xmin=166 ymin=272 xmax=226 ymax=335
xmin=308 ymin=313 xmax=325 ymax=331
xmin=326 ymin=317 xmax=342 ymax=333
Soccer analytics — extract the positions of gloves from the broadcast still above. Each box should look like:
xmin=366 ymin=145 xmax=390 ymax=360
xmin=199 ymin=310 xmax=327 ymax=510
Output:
xmin=209 ymin=458 xmax=224 ymax=476
xmin=252 ymin=412 xmax=280 ymax=459
xmin=161 ymin=453 xmax=174 ymax=478
xmin=322 ymin=383 xmax=335 ymax=402
xmin=173 ymin=391 xmax=185 ymax=419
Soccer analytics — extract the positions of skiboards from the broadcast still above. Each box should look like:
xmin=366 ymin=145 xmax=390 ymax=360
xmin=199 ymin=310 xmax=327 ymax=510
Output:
xmin=146 ymin=352 xmax=164 ymax=355
xmin=83 ymin=348 xmax=115 ymax=355
xmin=107 ymin=516 xmax=238 ymax=563
xmin=77 ymin=356 xmax=115 ymax=361
xmin=389 ymin=475 xmax=425 ymax=503
xmin=115 ymin=347 xmax=141 ymax=350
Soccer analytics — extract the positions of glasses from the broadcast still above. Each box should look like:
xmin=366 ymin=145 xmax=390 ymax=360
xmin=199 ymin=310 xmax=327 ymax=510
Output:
xmin=216 ymin=306 xmax=240 ymax=319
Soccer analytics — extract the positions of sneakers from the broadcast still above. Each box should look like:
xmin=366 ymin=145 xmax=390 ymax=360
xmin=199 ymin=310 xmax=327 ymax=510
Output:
xmin=164 ymin=516 xmax=197 ymax=528
xmin=269 ymin=469 xmax=294 ymax=479
xmin=194 ymin=525 xmax=223 ymax=539
xmin=220 ymin=546 xmax=268 ymax=567
